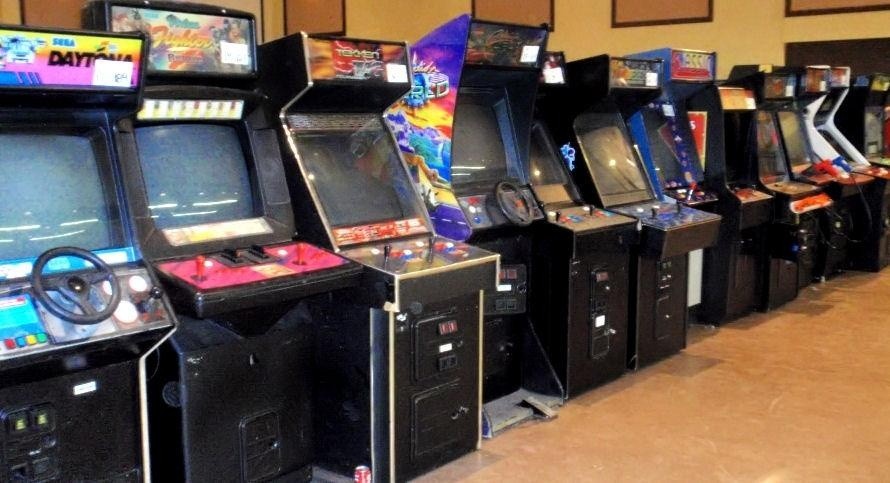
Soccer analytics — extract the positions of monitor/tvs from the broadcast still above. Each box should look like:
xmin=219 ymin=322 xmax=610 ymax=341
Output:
xmin=805 ymin=87 xmax=869 ymax=169
xmin=775 ymin=107 xmax=814 ymax=179
xmin=289 ymin=126 xmax=425 ymax=230
xmin=754 ymin=108 xmax=790 ymax=184
xmin=720 ymin=115 xmax=758 ymax=186
xmin=0 ymin=111 xmax=131 ymax=267
xmin=638 ymin=113 xmax=685 ymax=188
xmin=451 ymin=89 xmax=523 ymax=196
xmin=576 ymin=115 xmax=656 ymax=205
xmin=129 ymin=116 xmax=265 ymax=233
xmin=859 ymin=99 xmax=886 ymax=158
xmin=526 ymin=126 xmax=568 ymax=189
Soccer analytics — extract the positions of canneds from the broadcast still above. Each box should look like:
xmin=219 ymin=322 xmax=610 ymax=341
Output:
xmin=350 ymin=460 xmax=375 ymax=481
